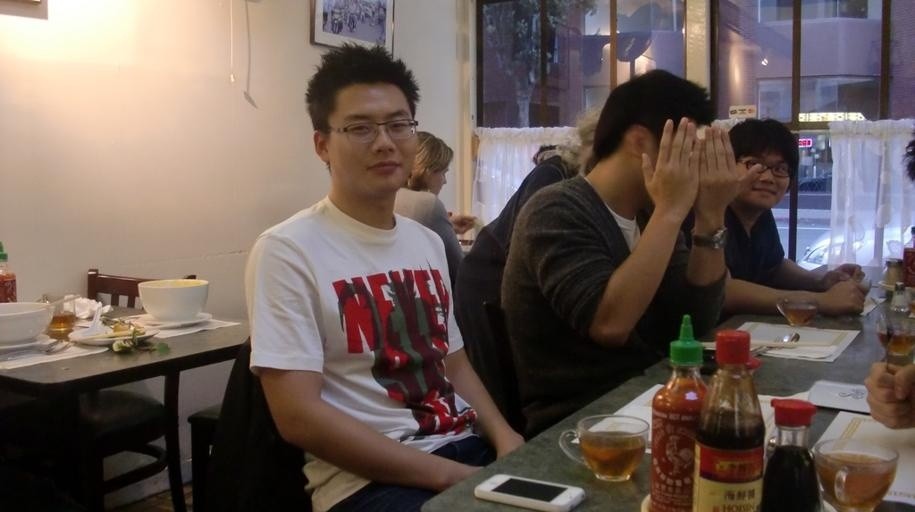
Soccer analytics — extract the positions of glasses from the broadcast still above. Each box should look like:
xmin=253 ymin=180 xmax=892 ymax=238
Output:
xmin=329 ymin=120 xmax=418 ymax=145
xmin=739 ymin=159 xmax=789 ymax=177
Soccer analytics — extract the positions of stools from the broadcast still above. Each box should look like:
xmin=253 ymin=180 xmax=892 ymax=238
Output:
xmin=188 ymin=403 xmax=221 ymax=512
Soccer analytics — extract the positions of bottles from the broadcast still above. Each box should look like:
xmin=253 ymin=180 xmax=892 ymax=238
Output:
xmin=885 ymin=258 xmax=904 ymax=298
xmin=651 ymin=313 xmax=825 ymax=512
xmin=0 ymin=242 xmax=17 ymax=302
xmin=902 ymin=226 xmax=915 ymax=286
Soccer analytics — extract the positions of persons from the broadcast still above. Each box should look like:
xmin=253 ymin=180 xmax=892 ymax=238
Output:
xmin=901 ymin=129 xmax=915 ymax=183
xmin=499 ymin=69 xmax=763 ymax=441
xmin=716 ymin=118 xmax=869 ymax=327
xmin=394 ymin=131 xmax=477 ymax=298
xmin=865 ymin=362 xmax=915 ymax=429
xmin=454 ymin=100 xmax=606 ymax=442
xmin=245 ymin=40 xmax=526 ymax=512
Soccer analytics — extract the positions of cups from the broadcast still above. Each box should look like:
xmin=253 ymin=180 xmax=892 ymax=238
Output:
xmin=876 ymin=284 xmax=915 ymax=375
xmin=41 ymin=290 xmax=75 ymax=331
xmin=559 ymin=412 xmax=650 ymax=483
xmin=775 ymin=295 xmax=820 ymax=327
xmin=813 ymin=437 xmax=901 ymax=511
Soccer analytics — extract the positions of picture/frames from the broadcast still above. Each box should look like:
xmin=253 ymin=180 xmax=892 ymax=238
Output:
xmin=308 ymin=1 xmax=397 ymax=62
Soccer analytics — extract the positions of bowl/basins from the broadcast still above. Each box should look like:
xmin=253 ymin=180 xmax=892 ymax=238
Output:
xmin=137 ymin=278 xmax=209 ymax=322
xmin=0 ymin=301 xmax=56 ymax=343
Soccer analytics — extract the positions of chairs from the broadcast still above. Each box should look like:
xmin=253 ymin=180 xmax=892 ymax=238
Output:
xmin=201 ymin=334 xmax=312 ymax=511
xmin=70 ymin=268 xmax=201 ymax=512
xmin=473 ymin=295 xmax=522 ymax=430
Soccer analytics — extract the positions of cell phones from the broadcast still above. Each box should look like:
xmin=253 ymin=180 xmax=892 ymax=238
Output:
xmin=474 ymin=472 xmax=585 ymax=512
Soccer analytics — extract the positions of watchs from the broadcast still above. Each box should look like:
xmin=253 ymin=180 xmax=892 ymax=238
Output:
xmin=691 ymin=224 xmax=730 ymax=250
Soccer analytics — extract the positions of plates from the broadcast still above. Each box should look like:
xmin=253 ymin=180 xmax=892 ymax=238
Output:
xmin=69 ymin=325 xmax=160 ymax=346
xmin=137 ymin=313 xmax=212 ymax=329
xmin=1 ymin=331 xmax=57 ymax=353
